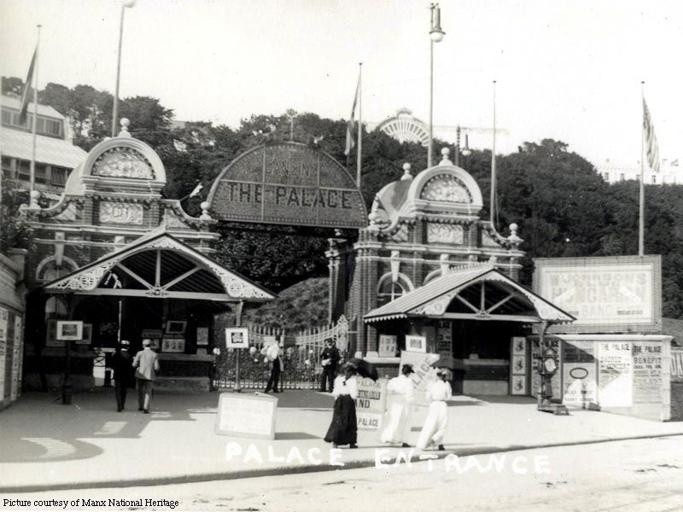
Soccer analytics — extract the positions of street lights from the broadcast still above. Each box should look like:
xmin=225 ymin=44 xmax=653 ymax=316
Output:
xmin=111 ymin=1 xmax=137 ymax=141
xmin=427 ymin=2 xmax=444 ymax=167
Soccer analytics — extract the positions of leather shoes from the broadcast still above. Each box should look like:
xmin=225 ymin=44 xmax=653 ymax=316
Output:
xmin=117 ymin=407 xmax=124 ymax=412
xmin=350 ymin=445 xmax=358 ymax=448
xmin=138 ymin=408 xmax=149 ymax=414
xmin=402 ymin=443 xmax=410 ymax=447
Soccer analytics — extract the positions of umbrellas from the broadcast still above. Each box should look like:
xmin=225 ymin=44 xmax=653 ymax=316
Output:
xmin=346 ymin=358 xmax=379 ymax=383
xmin=430 ymin=357 xmax=471 ymax=381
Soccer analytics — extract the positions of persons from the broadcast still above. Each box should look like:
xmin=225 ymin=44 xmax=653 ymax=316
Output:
xmin=131 ymin=339 xmax=160 ymax=414
xmin=265 ymin=335 xmax=284 ymax=393
xmin=324 ymin=366 xmax=358 ymax=448
xmin=109 ymin=340 xmax=131 ymax=412
xmin=416 ymin=372 xmax=452 ymax=451
xmin=209 ymin=347 xmax=221 ymax=392
xmin=318 ymin=338 xmax=340 ymax=392
xmin=380 ymin=364 xmax=416 ymax=447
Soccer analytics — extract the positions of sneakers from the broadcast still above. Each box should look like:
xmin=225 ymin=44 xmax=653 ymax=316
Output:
xmin=439 ymin=445 xmax=444 ymax=450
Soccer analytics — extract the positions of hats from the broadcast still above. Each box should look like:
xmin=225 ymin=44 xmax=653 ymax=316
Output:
xmin=121 ymin=340 xmax=130 ymax=345
xmin=142 ymin=339 xmax=152 ymax=345
